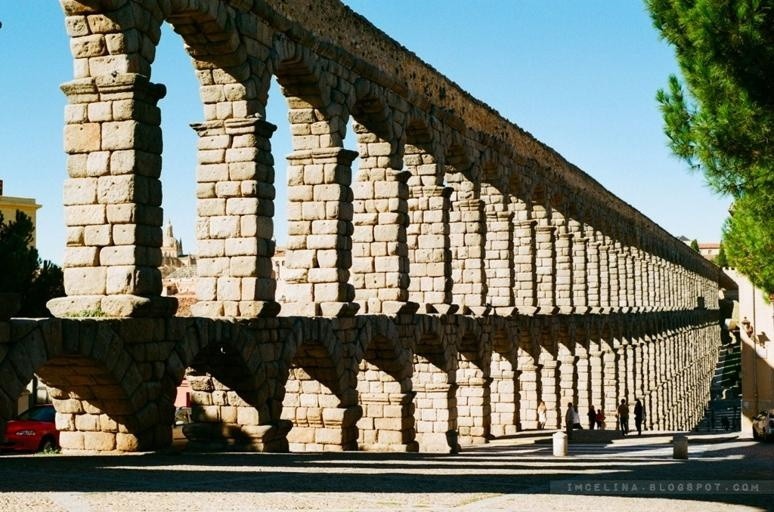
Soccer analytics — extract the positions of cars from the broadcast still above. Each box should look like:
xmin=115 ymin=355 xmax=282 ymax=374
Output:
xmin=749 ymin=408 xmax=774 ymax=441
xmin=2 ymin=404 xmax=59 ymax=453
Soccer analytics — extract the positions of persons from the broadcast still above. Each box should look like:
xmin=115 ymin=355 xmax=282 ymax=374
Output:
xmin=617 ymin=399 xmax=629 ymax=435
xmin=538 ymin=402 xmax=547 ymax=429
xmin=634 ymin=400 xmax=642 ymax=436
xmin=587 ymin=405 xmax=596 ymax=430
xmin=596 ymin=409 xmax=604 ymax=431
xmin=565 ymin=403 xmax=574 ymax=440
